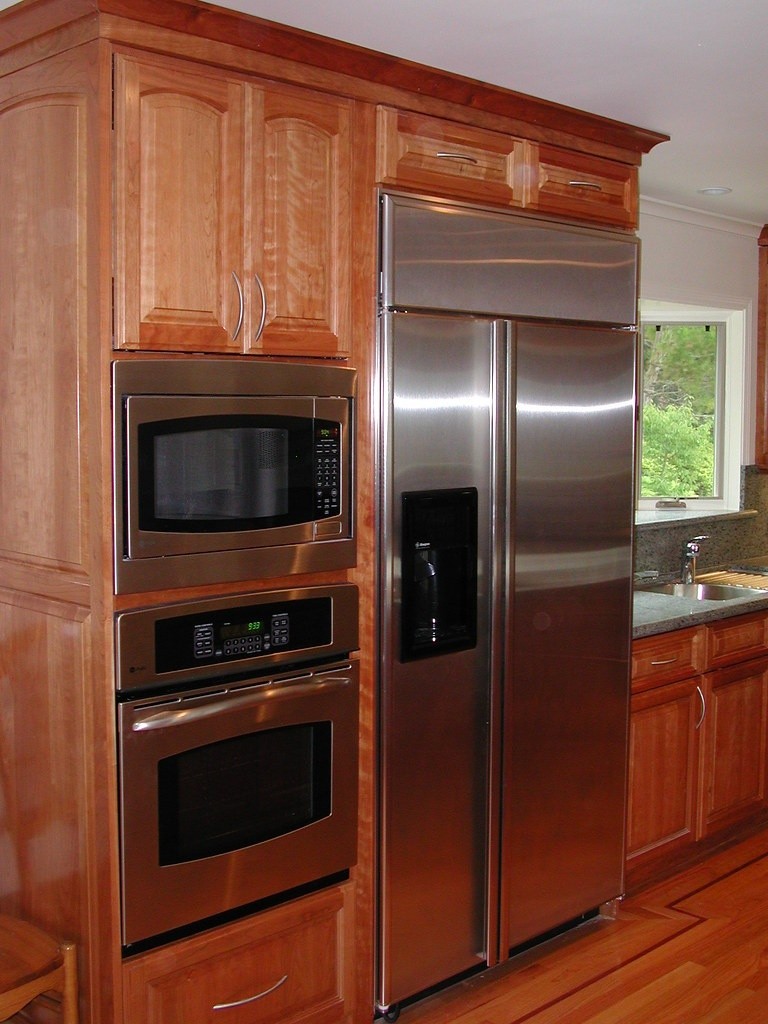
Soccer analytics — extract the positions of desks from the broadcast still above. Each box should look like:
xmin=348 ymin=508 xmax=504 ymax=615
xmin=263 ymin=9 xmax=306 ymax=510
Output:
xmin=0 ymin=911 xmax=80 ymax=1024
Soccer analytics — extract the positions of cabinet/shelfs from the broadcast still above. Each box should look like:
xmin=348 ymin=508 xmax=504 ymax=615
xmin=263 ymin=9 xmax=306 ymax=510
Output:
xmin=0 ymin=1 xmax=378 ymax=1024
xmin=375 ymin=50 xmax=671 ymax=226
xmin=625 ymin=609 xmax=768 ymax=895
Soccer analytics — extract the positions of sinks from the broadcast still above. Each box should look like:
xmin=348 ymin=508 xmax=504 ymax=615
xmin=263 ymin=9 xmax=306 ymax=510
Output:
xmin=634 ymin=579 xmax=767 ymax=603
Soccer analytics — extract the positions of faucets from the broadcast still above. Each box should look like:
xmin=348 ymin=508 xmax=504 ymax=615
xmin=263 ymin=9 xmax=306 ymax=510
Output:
xmin=681 ymin=534 xmax=710 ymax=584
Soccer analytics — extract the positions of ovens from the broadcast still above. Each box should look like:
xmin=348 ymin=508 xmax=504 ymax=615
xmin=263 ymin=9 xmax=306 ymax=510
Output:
xmin=115 ymin=585 xmax=360 ymax=952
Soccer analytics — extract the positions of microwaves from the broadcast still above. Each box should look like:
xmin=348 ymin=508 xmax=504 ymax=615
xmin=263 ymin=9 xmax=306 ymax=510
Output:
xmin=110 ymin=359 xmax=360 ymax=599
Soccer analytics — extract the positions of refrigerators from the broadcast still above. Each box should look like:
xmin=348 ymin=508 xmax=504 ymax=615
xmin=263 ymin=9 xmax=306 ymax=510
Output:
xmin=375 ymin=194 xmax=642 ymax=1016
